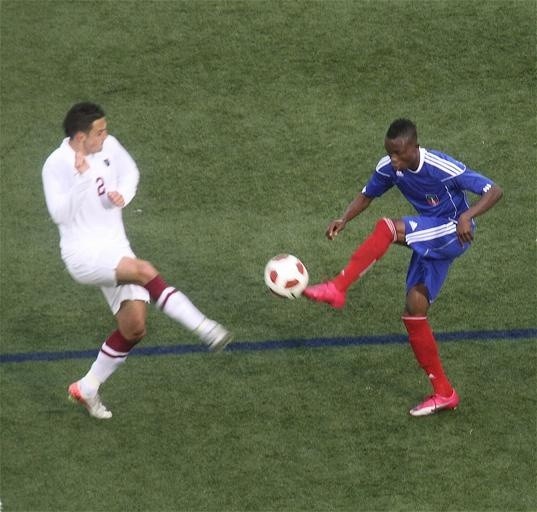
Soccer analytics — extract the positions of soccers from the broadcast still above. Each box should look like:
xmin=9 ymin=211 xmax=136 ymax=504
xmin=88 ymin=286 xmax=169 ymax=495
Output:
xmin=264 ymin=253 xmax=309 ymax=300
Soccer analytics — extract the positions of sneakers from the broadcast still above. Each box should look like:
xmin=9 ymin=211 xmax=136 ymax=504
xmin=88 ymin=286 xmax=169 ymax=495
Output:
xmin=194 ymin=318 xmax=232 ymax=352
xmin=303 ymin=282 xmax=345 ymax=308
xmin=410 ymin=388 xmax=458 ymax=416
xmin=69 ymin=379 xmax=112 ymax=419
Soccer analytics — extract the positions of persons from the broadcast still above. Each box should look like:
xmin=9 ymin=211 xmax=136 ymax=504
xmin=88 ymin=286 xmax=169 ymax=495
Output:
xmin=298 ymin=117 xmax=504 ymax=417
xmin=41 ymin=102 xmax=233 ymax=418
